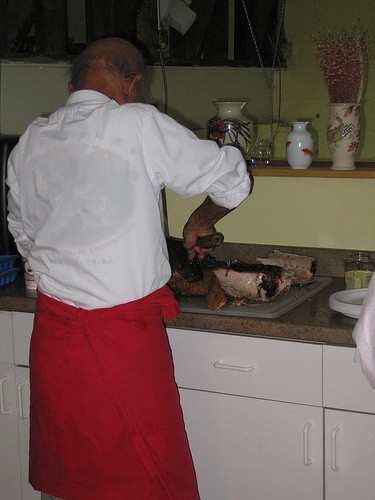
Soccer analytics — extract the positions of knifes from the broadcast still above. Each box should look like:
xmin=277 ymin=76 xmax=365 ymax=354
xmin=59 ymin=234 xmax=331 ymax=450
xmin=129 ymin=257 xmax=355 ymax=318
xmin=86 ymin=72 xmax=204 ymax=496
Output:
xmin=167 ymin=233 xmax=224 ymax=283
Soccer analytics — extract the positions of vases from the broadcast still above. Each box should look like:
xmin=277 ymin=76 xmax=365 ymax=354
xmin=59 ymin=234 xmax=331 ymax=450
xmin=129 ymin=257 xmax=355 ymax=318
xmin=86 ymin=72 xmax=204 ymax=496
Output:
xmin=206 ymin=100 xmax=254 ymax=159
xmin=326 ymin=102 xmax=362 ymax=171
xmin=285 ymin=121 xmax=317 ymax=170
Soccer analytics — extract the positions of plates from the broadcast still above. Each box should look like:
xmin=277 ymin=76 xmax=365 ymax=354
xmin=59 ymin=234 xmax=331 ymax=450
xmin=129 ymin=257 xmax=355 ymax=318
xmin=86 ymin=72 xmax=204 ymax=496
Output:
xmin=328 ymin=288 xmax=368 ymax=319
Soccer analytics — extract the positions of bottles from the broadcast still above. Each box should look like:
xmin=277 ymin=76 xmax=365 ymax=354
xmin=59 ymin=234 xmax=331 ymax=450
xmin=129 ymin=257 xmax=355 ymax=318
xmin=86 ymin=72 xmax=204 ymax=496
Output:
xmin=206 ymin=101 xmax=254 ymax=160
xmin=344 ymin=252 xmax=374 ymax=290
xmin=285 ymin=121 xmax=315 ymax=170
xmin=249 ymin=141 xmax=272 ymax=169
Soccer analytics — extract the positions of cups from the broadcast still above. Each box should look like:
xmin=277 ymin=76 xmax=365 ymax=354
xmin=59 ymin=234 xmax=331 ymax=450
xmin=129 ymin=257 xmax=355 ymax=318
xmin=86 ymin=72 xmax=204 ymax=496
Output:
xmin=20 ymin=255 xmax=37 ymax=290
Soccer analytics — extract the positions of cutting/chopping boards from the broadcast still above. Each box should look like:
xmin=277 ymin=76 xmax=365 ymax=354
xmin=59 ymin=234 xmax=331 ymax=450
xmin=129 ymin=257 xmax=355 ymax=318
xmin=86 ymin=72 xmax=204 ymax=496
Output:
xmin=176 ymin=276 xmax=332 ymax=318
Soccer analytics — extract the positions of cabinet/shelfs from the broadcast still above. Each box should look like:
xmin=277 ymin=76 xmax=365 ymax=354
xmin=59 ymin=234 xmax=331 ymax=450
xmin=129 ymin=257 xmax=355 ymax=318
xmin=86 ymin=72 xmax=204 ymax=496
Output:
xmin=166 ymin=326 xmax=375 ymax=500
xmin=0 ymin=308 xmax=41 ymax=500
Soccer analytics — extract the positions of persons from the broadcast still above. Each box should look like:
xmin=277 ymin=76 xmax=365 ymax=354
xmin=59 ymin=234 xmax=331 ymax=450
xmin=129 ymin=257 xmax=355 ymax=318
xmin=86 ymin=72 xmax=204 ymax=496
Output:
xmin=5 ymin=38 xmax=251 ymax=500
